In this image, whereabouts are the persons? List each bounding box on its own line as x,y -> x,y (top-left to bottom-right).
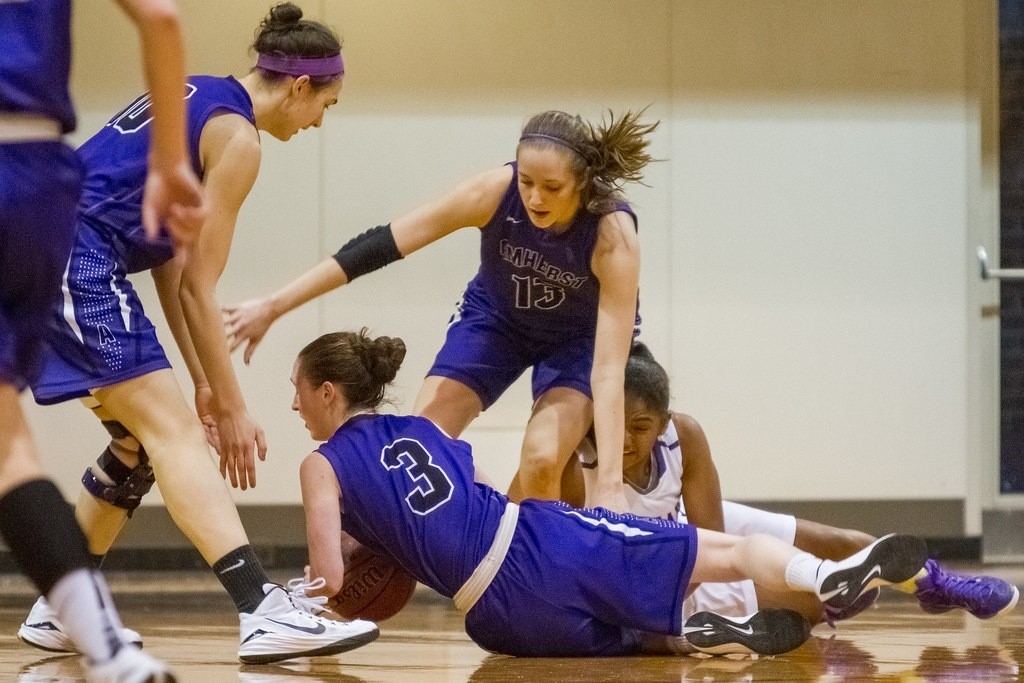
0,0 -> 207,683
220,112 -> 660,512
15,2 -> 380,664
508,343 -> 1021,624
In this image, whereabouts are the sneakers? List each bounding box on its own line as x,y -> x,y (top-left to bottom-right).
236,576 -> 379,665
76,643 -> 179,683
683,607 -> 810,660
16,592 -> 143,653
916,559 -> 1019,620
816,530 -> 929,619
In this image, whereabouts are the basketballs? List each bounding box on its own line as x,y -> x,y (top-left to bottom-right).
327,530 -> 418,620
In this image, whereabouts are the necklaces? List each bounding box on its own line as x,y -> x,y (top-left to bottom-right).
290,333 -> 928,657
